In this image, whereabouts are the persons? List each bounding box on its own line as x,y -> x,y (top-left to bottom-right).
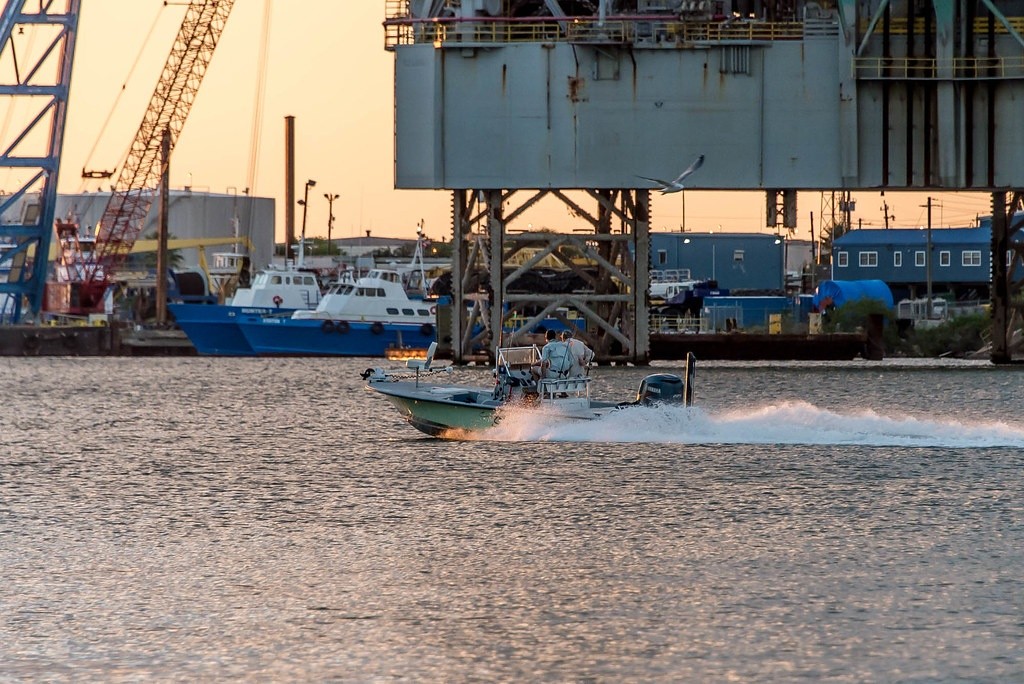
530,329 -> 595,399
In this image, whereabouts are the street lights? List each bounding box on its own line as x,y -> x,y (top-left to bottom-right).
296,179 -> 317,235
322,193 -> 340,257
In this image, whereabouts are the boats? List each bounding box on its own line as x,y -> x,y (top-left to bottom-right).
166,231 -> 325,356
228,220 -> 591,359
356,338 -> 686,434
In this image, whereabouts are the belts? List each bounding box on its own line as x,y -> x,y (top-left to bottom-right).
549,368 -> 568,373
